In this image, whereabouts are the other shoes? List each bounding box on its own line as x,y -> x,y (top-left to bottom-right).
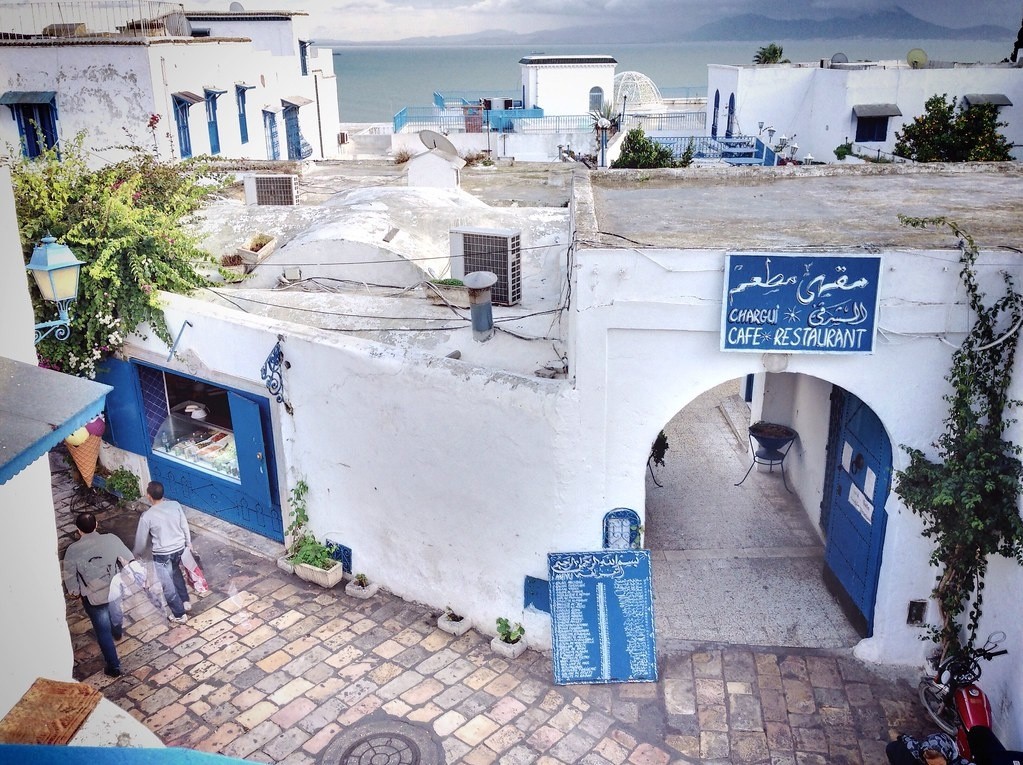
167,602 -> 193,624
104,631 -> 122,676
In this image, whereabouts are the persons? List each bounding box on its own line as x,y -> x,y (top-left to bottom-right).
131,481 -> 194,624
64,513 -> 145,675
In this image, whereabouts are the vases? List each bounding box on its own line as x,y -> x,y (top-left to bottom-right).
90,468 -> 115,492
747,421 -> 798,461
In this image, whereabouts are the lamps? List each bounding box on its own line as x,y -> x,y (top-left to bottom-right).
622,90 -> 629,97
757,119 -> 815,166
605,120 -> 611,129
23,237 -> 90,347
598,117 -> 607,130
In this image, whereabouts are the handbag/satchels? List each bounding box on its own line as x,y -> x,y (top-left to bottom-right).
182,547 -> 208,594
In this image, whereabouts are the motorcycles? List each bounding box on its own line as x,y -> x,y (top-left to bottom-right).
917,631 -> 1016,765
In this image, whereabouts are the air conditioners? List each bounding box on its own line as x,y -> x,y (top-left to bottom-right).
242,173 -> 301,207
340,131 -> 349,145
449,225 -> 524,306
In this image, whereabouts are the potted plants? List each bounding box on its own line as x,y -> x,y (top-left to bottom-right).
236,232 -> 278,265
61,450 -> 84,483
345,572 -> 378,599
292,531 -> 343,589
490,617 -> 528,659
219,253 -> 251,283
102,465 -> 143,510
437,605 -> 472,637
276,474 -> 315,574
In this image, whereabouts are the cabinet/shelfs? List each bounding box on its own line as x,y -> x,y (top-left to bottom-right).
152,412 -> 241,485
